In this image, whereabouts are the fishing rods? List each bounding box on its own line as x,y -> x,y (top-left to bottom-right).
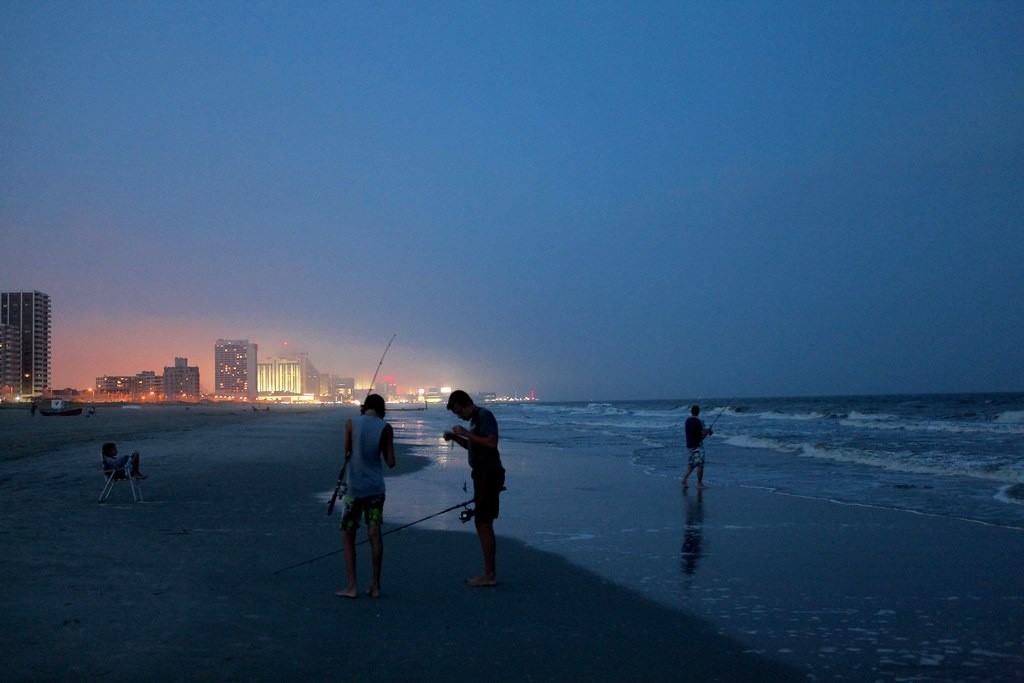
323,331 -> 399,517
705,392 -> 738,437
271,486 -> 510,576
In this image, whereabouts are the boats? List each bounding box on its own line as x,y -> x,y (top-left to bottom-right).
40,408 -> 82,416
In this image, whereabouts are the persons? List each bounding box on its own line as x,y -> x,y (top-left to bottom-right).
334,394 -> 396,597
31,404 -> 37,414
443,390 -> 506,586
681,405 -> 713,489
102,443 -> 148,479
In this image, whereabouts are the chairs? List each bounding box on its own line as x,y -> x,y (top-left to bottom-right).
98,443 -> 144,503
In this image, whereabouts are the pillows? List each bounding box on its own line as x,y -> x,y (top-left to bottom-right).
105,455 -> 129,468
125,448 -> 139,477
